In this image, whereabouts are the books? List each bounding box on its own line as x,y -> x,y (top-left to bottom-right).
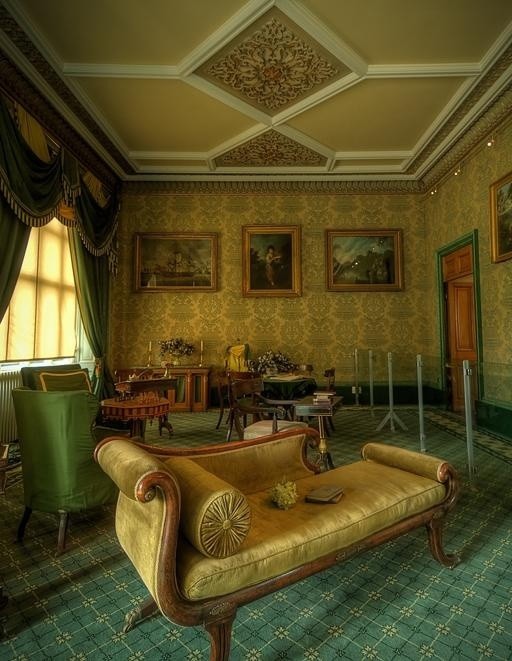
312,390 -> 338,405
328,491 -> 343,504
303,483 -> 344,503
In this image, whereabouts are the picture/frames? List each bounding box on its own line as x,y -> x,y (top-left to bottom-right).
488,169 -> 512,266
323,227 -> 409,296
239,224 -> 304,300
131,229 -> 220,295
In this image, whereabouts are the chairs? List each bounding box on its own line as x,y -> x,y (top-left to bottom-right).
10,364 -> 126,556
211,362 -> 338,442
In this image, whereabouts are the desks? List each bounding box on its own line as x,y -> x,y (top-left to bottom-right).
291,394 -> 346,472
99,378 -> 181,445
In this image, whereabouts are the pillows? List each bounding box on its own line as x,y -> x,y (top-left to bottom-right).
160,455 -> 253,561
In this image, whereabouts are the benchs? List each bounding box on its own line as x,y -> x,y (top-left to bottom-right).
89,428 -> 465,661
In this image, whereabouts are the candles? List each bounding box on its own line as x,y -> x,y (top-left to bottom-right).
200,340 -> 203,352
149,341 -> 152,352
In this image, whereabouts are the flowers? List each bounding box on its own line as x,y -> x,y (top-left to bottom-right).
247,351 -> 295,373
157,337 -> 196,360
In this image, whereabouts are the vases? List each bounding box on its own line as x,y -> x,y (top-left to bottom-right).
172,357 -> 181,366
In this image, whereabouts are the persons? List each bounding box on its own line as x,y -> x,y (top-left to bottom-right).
265,244 -> 282,288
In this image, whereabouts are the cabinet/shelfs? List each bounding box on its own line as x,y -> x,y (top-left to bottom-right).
131,366 -> 212,414
